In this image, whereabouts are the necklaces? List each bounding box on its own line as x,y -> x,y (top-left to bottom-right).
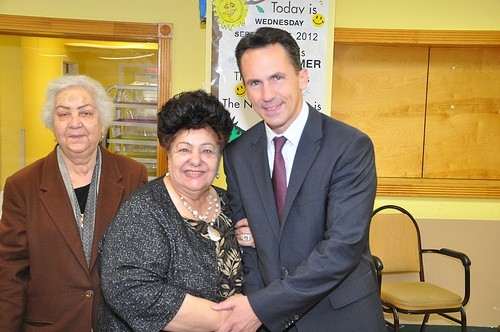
169,176 -> 219,226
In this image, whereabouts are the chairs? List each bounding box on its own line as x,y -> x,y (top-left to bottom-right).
367,204 -> 471,332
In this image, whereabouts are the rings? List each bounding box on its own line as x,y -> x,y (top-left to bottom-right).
243,233 -> 249,240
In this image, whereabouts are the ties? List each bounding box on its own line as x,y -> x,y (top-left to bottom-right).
272,137 -> 287,224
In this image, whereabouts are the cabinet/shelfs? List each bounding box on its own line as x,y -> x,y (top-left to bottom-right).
101,86 -> 157,182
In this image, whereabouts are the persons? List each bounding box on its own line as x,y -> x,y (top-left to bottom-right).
210,27 -> 388,332
96,89 -> 256,332
0,75 -> 148,332
